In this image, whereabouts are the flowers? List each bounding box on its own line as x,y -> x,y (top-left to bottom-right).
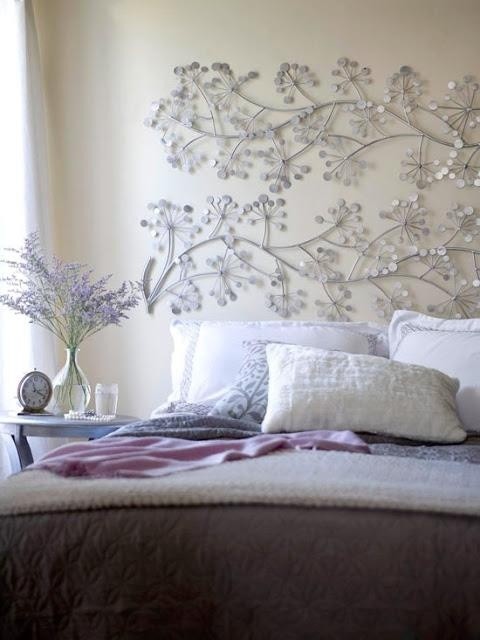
0,232 -> 142,347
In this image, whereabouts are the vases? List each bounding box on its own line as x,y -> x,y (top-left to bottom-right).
53,347 -> 91,412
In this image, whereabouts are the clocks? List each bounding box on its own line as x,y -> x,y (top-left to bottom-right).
17,368 -> 53,417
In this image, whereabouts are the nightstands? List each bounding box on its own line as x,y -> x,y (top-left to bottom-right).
1,413 -> 139,472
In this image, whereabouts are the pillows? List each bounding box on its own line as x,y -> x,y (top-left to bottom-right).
388,310 -> 480,432
149,318 -> 387,419
208,340 -> 283,424
261,343 -> 466,446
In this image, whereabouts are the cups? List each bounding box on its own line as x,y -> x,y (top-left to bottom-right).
96,380 -> 120,421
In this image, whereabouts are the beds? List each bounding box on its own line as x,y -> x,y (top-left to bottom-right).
0,412 -> 480,640
